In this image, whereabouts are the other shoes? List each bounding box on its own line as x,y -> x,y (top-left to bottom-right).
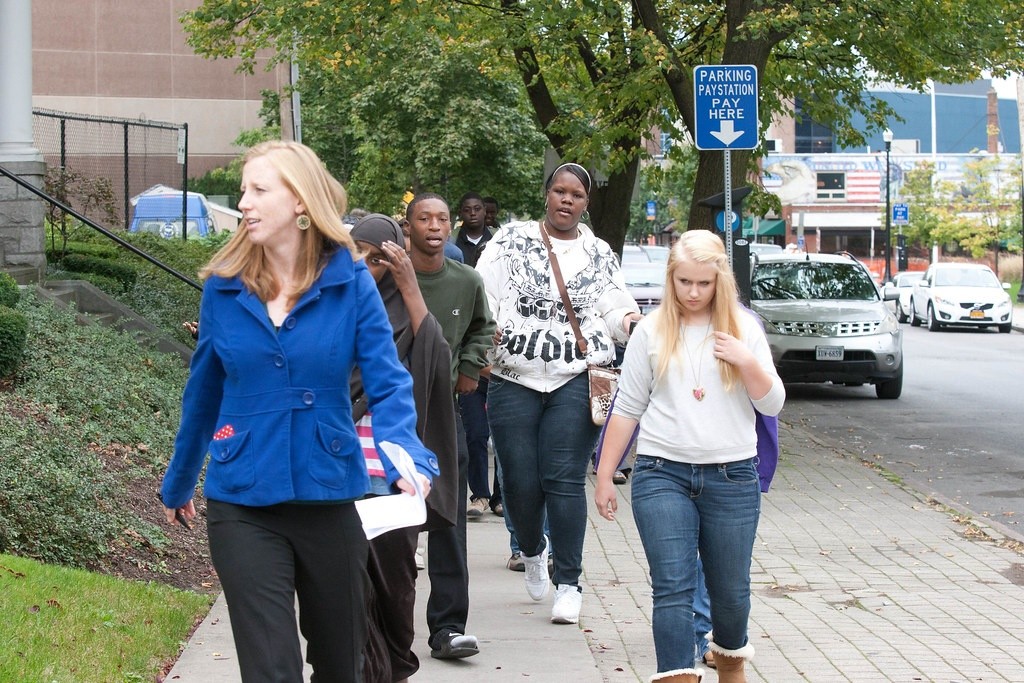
414,552 -> 426,569
612,470 -> 626,484
704,651 -> 716,668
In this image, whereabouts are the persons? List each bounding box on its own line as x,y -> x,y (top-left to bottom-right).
404,195 -> 498,658
398,191 -> 506,518
156,141 -> 437,683
594,228 -> 787,683
476,162 -> 648,623
595,303 -> 778,671
181,212 -> 454,683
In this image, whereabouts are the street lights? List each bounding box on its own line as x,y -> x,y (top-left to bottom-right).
882,127 -> 893,286
924,79 -> 938,263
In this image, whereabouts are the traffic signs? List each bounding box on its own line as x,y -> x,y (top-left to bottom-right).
693,65 -> 759,150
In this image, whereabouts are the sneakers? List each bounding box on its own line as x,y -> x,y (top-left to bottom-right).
494,503 -> 504,517
550,583 -> 582,624
467,496 -> 490,518
548,552 -> 555,579
520,534 -> 549,601
506,554 -> 525,572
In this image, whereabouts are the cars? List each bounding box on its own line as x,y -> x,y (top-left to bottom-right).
909,262 -> 1013,333
878,272 -> 926,323
749,244 -> 782,255
620,245 -> 673,316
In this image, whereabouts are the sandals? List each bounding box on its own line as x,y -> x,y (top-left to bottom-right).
430,629 -> 479,660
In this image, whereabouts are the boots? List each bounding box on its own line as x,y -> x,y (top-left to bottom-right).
705,628 -> 755,683
649,667 -> 705,683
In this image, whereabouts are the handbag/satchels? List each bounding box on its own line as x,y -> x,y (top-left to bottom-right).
587,364 -> 621,426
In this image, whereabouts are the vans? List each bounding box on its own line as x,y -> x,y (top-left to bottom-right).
131,191 -> 218,236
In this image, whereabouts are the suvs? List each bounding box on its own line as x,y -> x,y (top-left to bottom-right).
749,251 -> 904,398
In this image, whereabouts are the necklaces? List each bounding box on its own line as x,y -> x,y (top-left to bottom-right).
542,217 -> 579,259
679,305 -> 712,403
